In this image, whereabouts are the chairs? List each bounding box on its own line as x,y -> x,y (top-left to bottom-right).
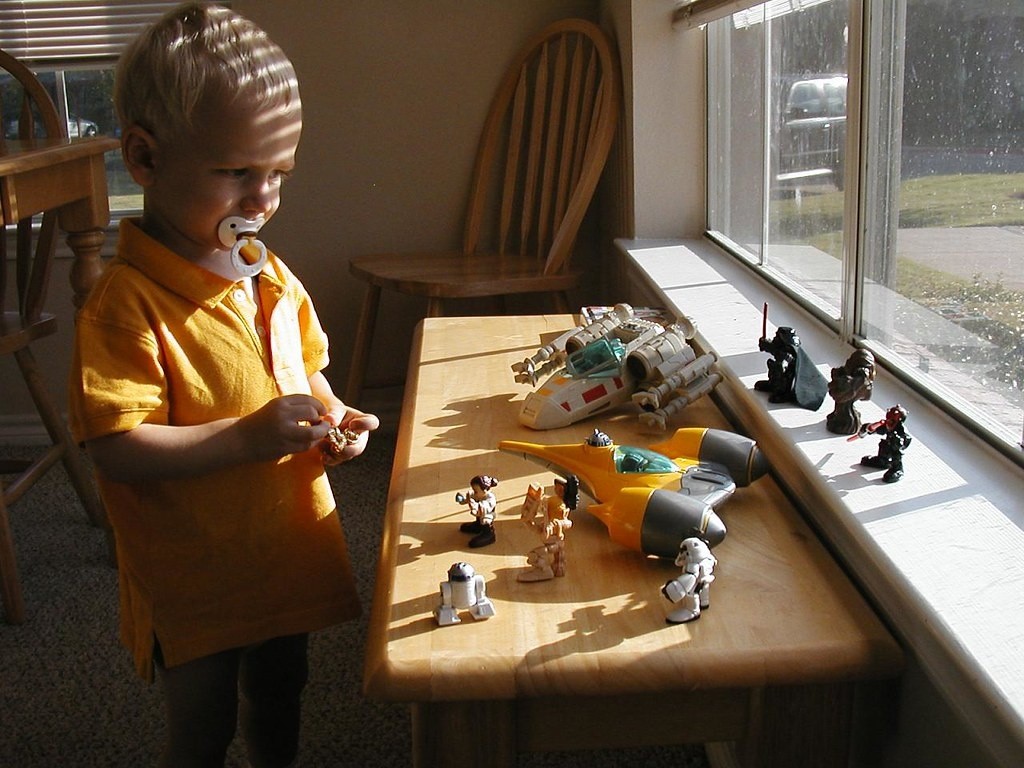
345,19 -> 618,437
0,48 -> 125,624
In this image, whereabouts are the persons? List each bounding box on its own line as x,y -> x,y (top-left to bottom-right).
455,475 -> 498,548
517,474 -> 579,582
754,299 -> 913,482
661,537 -> 717,625
63,0 -> 381,768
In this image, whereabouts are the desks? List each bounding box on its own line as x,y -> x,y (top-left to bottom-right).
0,132 -> 122,310
366,314 -> 896,768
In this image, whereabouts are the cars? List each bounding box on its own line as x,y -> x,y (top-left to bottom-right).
776,76 -> 851,188
6,111 -> 103,140
